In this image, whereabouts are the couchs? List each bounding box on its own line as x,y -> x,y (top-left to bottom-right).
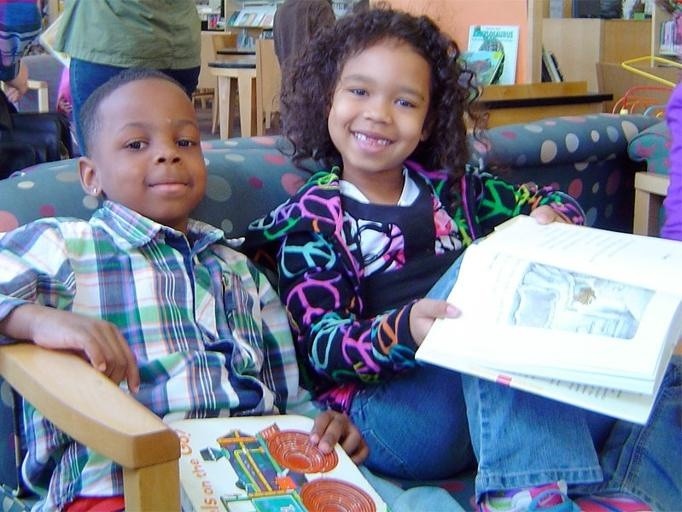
1,112 -> 658,512
13,55 -> 66,114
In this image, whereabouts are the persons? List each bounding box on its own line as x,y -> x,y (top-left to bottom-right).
244,10 -> 681,512
273,1 -> 336,68
0,0 -> 201,179
660,84 -> 682,239
0,70 -> 461,511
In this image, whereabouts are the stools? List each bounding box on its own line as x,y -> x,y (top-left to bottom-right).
208,60 -> 259,140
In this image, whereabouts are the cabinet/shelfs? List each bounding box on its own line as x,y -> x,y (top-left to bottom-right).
217,0 -> 282,132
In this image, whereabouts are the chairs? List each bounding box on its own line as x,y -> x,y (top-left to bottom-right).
1,83 -> 66,179
629,119 -> 674,237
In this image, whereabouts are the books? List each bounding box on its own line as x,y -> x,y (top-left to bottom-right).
416,210 -> 682,426
163,414 -> 388,511
456,25 -> 519,101
